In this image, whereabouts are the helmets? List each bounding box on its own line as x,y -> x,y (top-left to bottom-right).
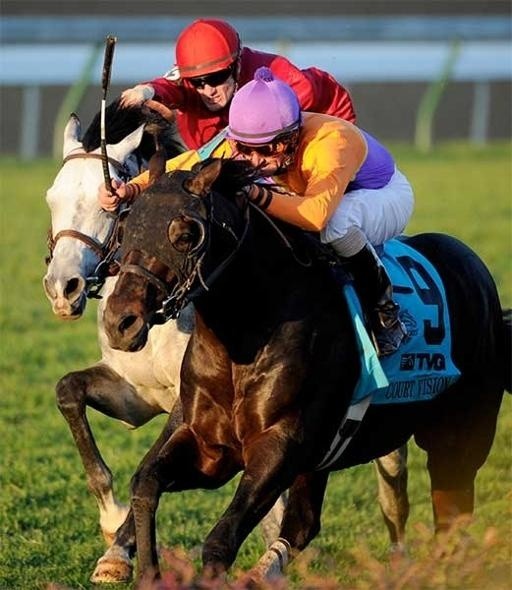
175,15 -> 242,78
224,67 -> 302,149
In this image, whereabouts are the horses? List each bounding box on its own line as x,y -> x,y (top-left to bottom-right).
41,113 -> 412,548
100,148 -> 512,589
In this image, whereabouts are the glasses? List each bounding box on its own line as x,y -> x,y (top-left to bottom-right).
235,145 -> 274,158
188,65 -> 235,90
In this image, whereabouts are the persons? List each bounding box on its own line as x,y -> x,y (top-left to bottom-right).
96,64 -> 416,359
115,11 -> 360,154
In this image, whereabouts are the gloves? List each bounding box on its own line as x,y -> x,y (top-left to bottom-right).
119,83 -> 157,107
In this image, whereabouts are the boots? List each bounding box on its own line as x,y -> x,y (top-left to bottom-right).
342,241 -> 409,357
